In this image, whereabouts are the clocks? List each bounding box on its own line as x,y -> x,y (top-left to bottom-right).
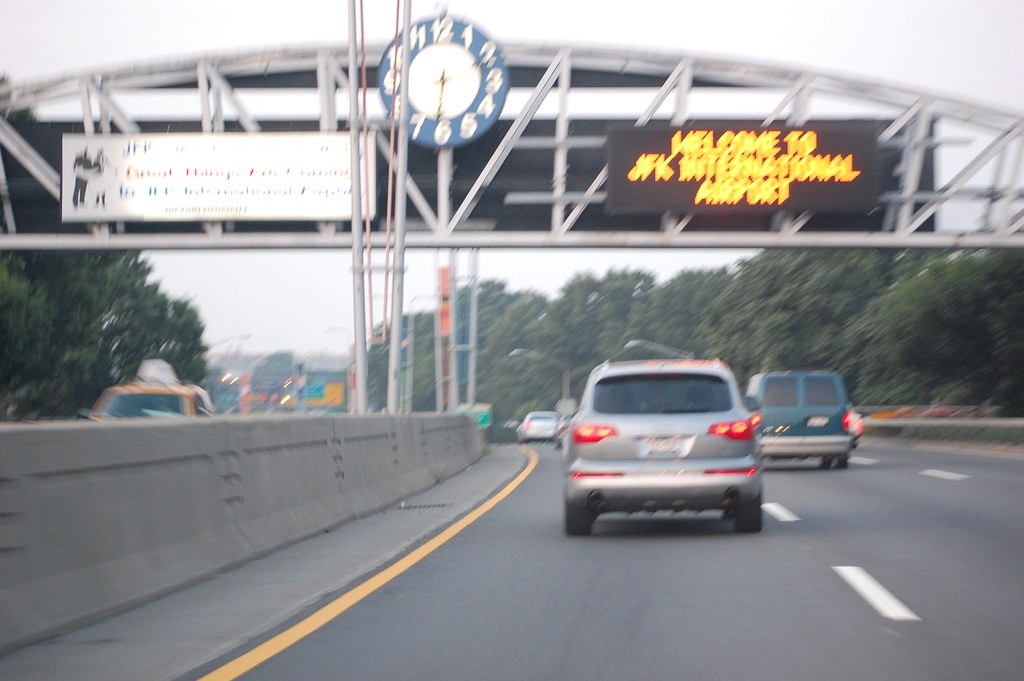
375,17 -> 511,150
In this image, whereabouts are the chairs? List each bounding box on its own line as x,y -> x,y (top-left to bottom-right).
600,384 -> 642,414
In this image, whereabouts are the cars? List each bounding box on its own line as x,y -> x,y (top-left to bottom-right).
743,369 -> 864,471
76,358 -> 217,423
516,410 -> 559,444
553,355 -> 766,537
